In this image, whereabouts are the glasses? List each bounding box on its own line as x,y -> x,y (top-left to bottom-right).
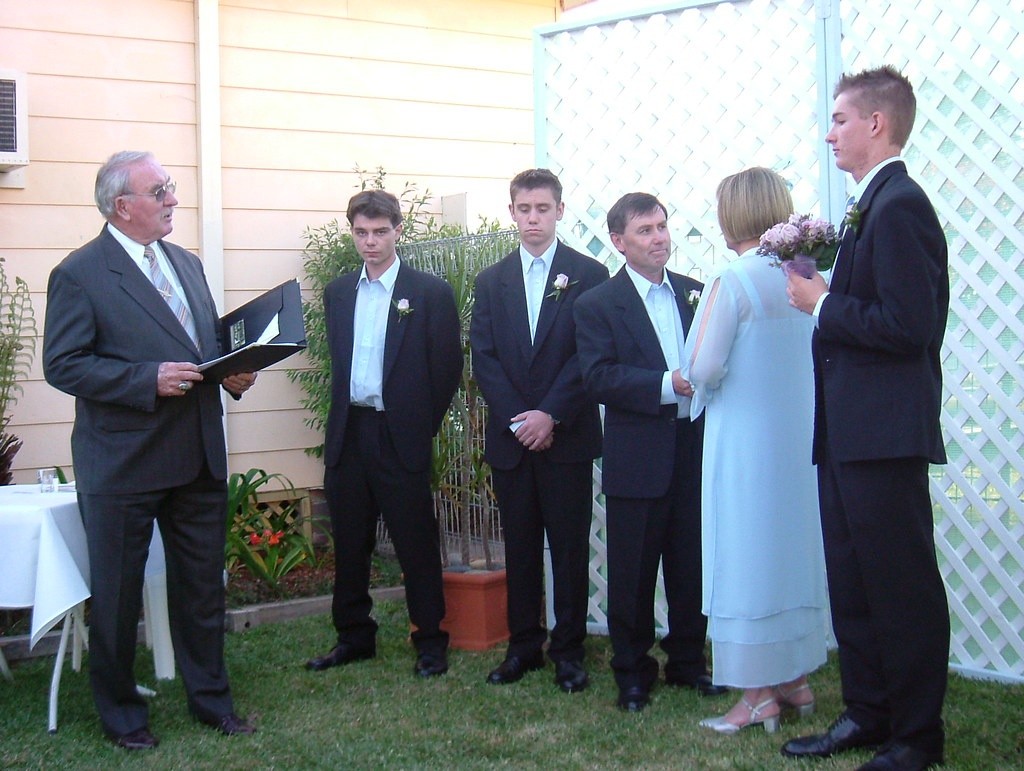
121,182 -> 176,203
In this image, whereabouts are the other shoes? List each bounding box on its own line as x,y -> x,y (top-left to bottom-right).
110,727 -> 158,752
200,713 -> 256,739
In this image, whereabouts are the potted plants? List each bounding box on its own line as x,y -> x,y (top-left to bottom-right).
402,244 -> 510,650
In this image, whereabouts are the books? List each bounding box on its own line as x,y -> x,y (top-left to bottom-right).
196,278 -> 308,384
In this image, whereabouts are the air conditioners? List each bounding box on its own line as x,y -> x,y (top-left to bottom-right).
0,72 -> 30,173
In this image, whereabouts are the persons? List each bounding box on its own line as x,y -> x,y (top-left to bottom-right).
303,190 -> 463,675
43,151 -> 257,750
470,168 -> 609,691
782,66 -> 951,771
573,192 -> 727,711
677,167 -> 837,735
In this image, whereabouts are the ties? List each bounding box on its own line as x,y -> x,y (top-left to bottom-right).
144,246 -> 203,357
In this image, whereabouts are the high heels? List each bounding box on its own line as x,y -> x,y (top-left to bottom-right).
775,682 -> 814,719
699,696 -> 780,734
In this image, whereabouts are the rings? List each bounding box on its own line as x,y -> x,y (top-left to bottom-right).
179,382 -> 189,391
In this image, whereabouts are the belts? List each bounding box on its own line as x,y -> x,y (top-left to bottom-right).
349,405 -> 385,416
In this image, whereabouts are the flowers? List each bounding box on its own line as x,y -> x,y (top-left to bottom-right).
684,288 -> 701,313
845,203 -> 861,233
546,273 -> 580,301
391,298 -> 414,323
755,213 -> 842,279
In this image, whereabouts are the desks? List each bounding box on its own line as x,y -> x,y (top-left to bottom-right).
0,485 -> 175,734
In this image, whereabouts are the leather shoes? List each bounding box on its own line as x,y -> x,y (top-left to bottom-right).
414,646 -> 448,679
305,643 -> 376,671
486,653 -> 545,685
665,669 -> 725,696
779,711 -> 890,759
555,658 -> 588,694
853,736 -> 944,771
618,683 -> 648,713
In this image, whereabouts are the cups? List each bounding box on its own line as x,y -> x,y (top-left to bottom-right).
38,468 -> 59,493
